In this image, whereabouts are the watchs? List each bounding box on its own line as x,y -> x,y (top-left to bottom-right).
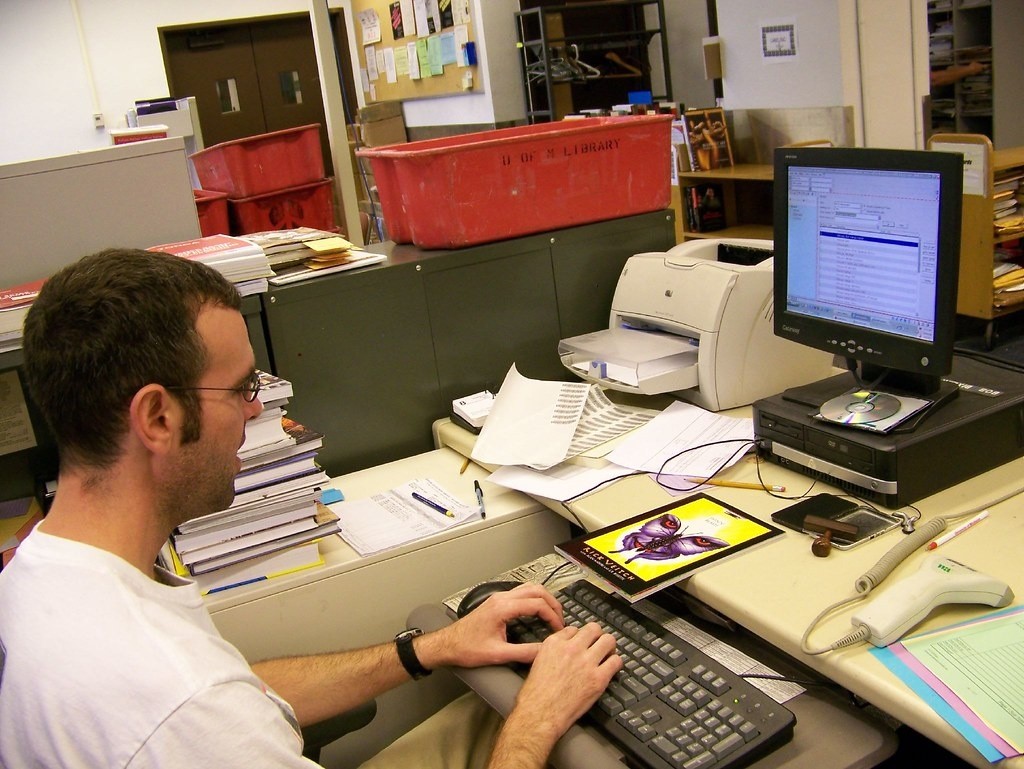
395,628 -> 433,681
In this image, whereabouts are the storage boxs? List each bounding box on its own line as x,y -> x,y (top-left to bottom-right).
187,123 -> 336,239
348,101 -> 674,251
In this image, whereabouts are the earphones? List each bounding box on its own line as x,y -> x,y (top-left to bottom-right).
892,511 -> 910,527
902,516 -> 918,535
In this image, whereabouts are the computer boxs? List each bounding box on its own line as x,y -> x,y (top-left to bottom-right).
752,353 -> 1024,510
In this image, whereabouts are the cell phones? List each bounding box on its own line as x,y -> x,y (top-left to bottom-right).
769,493 -> 859,532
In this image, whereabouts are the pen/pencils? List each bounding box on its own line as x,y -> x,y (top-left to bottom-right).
924,509 -> 991,552
474,479 -> 488,519
411,491 -> 456,519
682,476 -> 786,492
457,456 -> 473,476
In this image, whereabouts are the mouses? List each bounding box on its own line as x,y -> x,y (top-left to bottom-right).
457,581 -> 524,619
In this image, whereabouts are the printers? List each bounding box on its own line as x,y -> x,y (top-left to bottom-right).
558,237 -> 849,412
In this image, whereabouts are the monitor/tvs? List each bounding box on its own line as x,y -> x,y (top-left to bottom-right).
771,146 -> 964,432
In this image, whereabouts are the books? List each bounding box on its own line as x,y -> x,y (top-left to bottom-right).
148,226 -> 388,296
929,0 -> 992,119
993,262 -> 1020,277
992,181 -> 1019,219
554,494 -> 786,603
169,367 -> 341,594
109,125 -> 167,145
0,279 -> 49,354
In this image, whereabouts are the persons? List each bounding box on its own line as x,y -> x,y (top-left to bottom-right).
0,247 -> 621,769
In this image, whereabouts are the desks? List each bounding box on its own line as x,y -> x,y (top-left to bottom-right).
0,206 -> 1024,767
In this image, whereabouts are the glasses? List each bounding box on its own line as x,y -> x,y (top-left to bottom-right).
128,370 -> 263,402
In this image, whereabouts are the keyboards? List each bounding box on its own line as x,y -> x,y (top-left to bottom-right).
505,578 -> 797,769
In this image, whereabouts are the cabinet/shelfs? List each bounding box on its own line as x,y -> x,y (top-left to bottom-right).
927,0 -> 1024,150
674,172 -> 777,249
928,133 -> 1024,349
513,0 -> 674,124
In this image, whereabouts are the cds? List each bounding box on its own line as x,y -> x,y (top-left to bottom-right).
819,392 -> 901,423
